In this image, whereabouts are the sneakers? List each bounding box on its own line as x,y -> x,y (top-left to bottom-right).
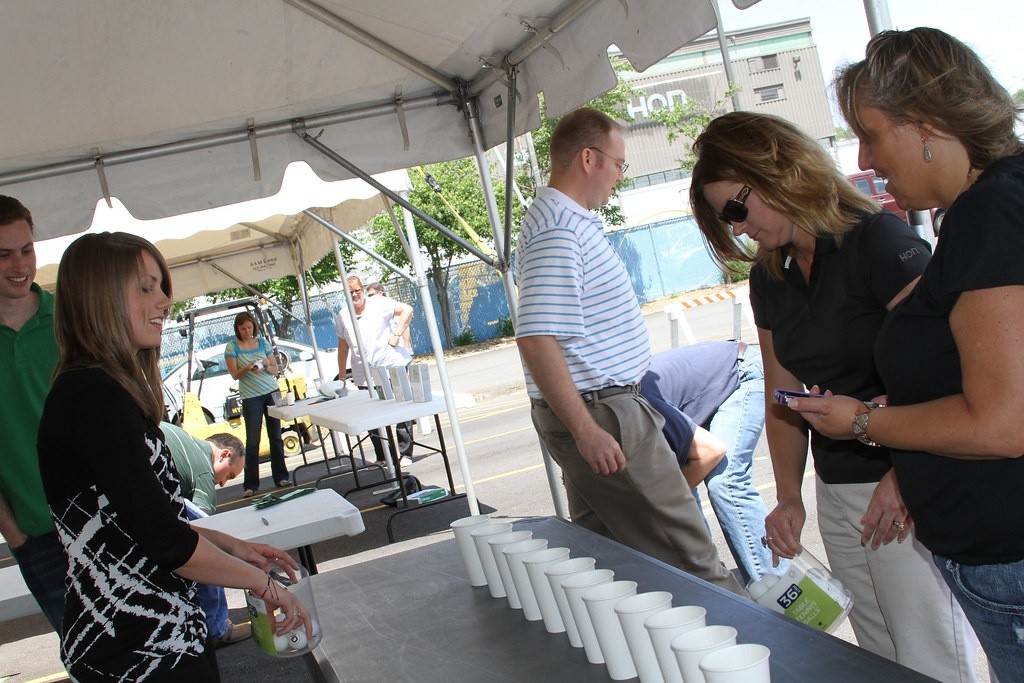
212,619 -> 252,649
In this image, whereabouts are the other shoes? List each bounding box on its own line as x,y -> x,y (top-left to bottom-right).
277,480 -> 291,487
243,488 -> 256,498
399,455 -> 412,467
374,460 -> 387,468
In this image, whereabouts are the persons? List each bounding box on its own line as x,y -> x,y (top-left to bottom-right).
36,231 -> 313,683
790,27 -> 1024,683
366,282 -> 414,356
514,107 -> 746,597
335,276 -> 413,467
158,421 -> 253,648
688,111 -> 991,683
0,194 -> 65,639
224,312 -> 292,497
636,339 -> 790,587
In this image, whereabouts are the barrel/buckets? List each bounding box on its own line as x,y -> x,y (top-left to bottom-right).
743,544 -> 856,635
243,562 -> 322,657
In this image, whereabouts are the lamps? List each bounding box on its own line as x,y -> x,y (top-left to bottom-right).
793,55 -> 800,70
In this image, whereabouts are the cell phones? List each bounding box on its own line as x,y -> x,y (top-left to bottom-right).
771,389 -> 825,406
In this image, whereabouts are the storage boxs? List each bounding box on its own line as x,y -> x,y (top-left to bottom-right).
396,489 -> 448,508
371,364 -> 432,403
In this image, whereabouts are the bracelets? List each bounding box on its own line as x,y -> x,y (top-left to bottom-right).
394,334 -> 401,336
248,573 -> 279,600
852,401 -> 885,447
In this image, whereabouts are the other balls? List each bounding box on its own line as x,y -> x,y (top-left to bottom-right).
272,613 -> 319,652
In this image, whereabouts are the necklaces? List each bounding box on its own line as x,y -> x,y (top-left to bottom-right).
933,166 -> 973,238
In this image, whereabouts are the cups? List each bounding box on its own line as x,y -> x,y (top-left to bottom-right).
314,377 -> 325,395
450,515 -> 491,587
545,557 -> 596,648
470,523 -> 514,598
671,625 -> 737,683
522,547 -> 570,633
698,643 -> 771,683
614,592 -> 673,683
644,606 -> 706,683
582,580 -> 638,680
488,531 -> 533,608
503,539 -> 548,621
561,570 -> 614,664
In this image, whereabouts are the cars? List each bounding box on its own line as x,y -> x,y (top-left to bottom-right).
159,337 -> 367,429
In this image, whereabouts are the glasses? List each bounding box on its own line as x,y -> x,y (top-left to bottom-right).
350,288 -> 362,295
368,292 -> 379,297
719,184 -> 752,226
586,146 -> 629,173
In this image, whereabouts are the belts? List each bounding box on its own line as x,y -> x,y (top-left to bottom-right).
735,341 -> 747,366
534,382 -> 641,408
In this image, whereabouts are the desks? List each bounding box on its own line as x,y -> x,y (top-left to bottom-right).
286,515 -> 944,683
0,488 -> 366,623
309,390 -> 482,544
268,391 -> 387,490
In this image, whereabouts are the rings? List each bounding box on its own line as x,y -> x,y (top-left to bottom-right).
761,536 -> 772,549
893,521 -> 905,530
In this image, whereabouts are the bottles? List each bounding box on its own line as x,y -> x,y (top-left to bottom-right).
251,361 -> 262,371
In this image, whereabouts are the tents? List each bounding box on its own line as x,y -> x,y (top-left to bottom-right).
0,0 -> 762,523
33,161 -> 480,517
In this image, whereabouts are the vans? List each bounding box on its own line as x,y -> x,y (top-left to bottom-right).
841,169 -> 946,239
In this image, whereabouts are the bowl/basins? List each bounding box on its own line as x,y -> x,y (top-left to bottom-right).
271,389 -> 295,407
319,381 -> 344,397
